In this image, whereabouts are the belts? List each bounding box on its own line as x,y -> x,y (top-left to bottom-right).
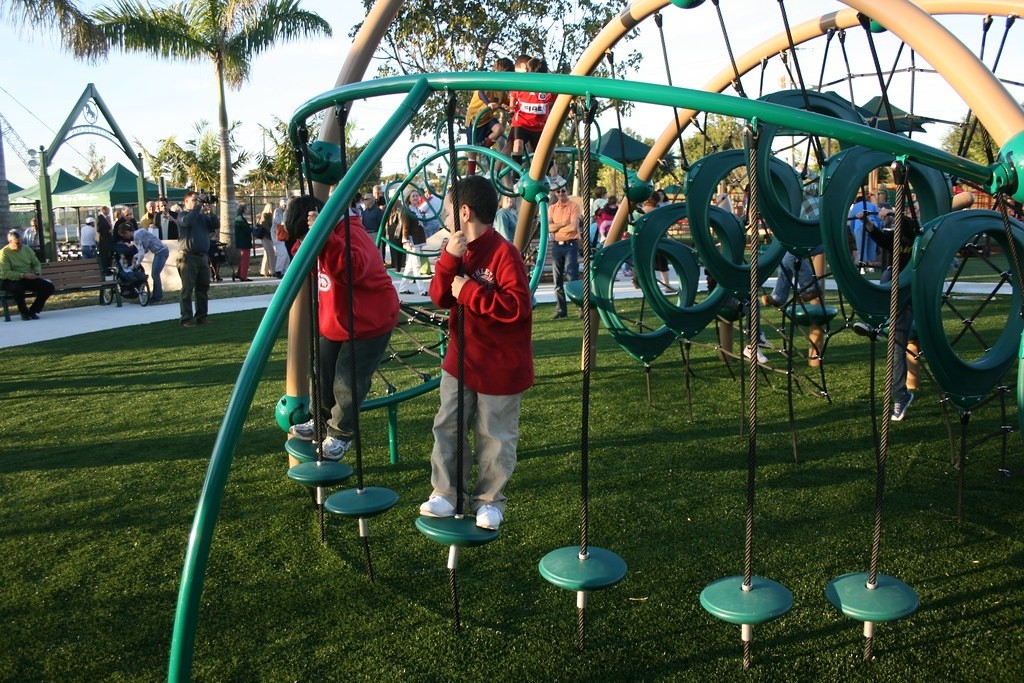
555,239 -> 577,246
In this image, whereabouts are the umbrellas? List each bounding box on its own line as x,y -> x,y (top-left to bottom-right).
858,96 -> 928,134
579,129 -> 670,199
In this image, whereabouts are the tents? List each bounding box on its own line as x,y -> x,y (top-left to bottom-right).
7,161 -> 195,223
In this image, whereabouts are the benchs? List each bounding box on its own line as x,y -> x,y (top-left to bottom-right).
526,238 -> 585,283
0,257 -> 123,322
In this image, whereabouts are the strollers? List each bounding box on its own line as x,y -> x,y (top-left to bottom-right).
208,236 -> 236,282
102,242 -> 150,306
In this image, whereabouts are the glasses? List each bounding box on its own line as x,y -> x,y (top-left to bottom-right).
556,190 -> 566,193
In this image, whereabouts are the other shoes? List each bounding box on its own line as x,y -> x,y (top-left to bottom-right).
201,319 -> 210,323
421,291 -> 429,296
548,310 -> 567,320
21,310 -> 29,320
800,291 -> 817,304
149,297 -> 156,302
400,290 -> 415,294
28,310 -> 39,319
577,309 -> 584,318
183,321 -> 196,327
762,295 -> 780,307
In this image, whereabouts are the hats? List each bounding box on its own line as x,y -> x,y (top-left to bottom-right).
86,217 -> 95,223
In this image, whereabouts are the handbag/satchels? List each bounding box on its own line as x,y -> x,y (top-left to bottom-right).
419,246 -> 431,274
252,224 -> 266,239
277,225 -> 289,241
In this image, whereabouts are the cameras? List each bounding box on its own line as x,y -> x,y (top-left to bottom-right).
201,189 -> 218,203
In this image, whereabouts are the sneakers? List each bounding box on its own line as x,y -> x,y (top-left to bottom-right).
475,504 -> 503,530
289,419 -> 327,440
758,335 -> 771,348
420,496 -> 456,518
891,393 -> 914,421
315,437 -> 351,459
743,346 -> 768,363
853,322 -> 883,341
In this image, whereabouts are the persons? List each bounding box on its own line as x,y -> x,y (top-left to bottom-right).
418,174 -> 534,530
711,164 -> 976,420
81,54 -> 682,321
175,190 -> 221,328
0,228 -> 55,321
283,194 -> 400,461
